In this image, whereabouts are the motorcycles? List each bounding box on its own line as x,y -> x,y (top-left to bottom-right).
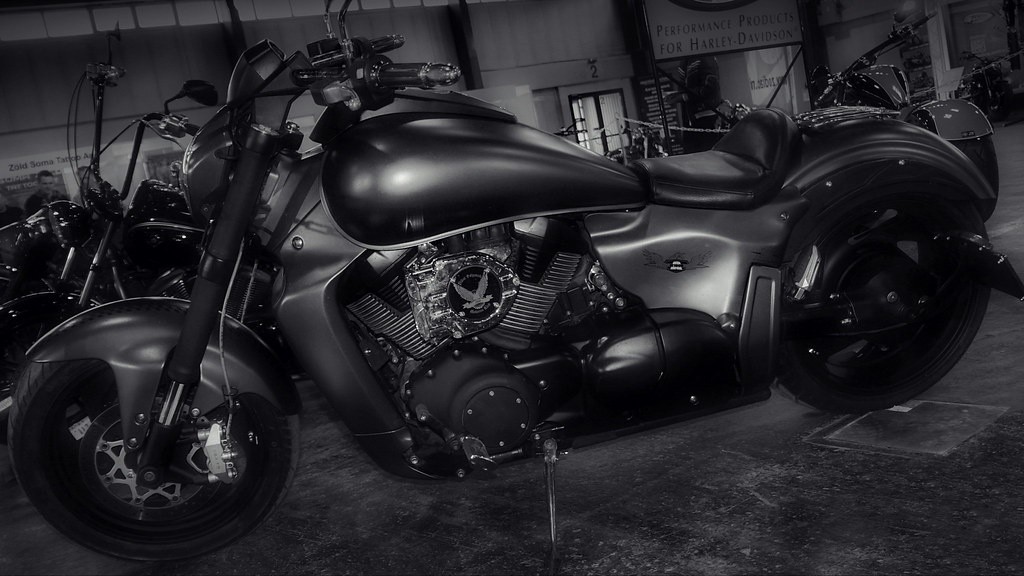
0,23 -> 1024,460
8,1 -> 1023,562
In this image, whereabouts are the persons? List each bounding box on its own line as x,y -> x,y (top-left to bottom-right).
0,166 -> 104,229
665,55 -> 722,154
